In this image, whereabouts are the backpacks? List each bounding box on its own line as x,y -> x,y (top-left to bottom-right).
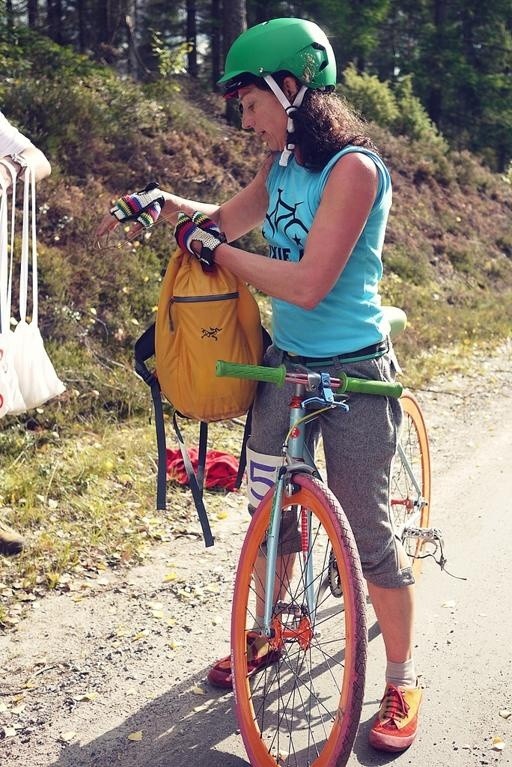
154,236 -> 265,421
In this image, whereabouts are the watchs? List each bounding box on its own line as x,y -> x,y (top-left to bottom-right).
3,153 -> 29,178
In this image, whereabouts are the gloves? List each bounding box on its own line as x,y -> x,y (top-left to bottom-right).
110,182 -> 167,231
168,207 -> 230,269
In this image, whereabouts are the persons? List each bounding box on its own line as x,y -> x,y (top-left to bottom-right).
96,18 -> 422,752
0,109 -> 51,554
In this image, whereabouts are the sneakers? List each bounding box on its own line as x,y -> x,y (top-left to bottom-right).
209,625 -> 276,693
367,677 -> 425,754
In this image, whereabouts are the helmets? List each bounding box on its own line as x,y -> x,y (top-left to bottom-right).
211,17 -> 338,97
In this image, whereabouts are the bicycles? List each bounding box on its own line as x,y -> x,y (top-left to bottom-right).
214,305 -> 467,767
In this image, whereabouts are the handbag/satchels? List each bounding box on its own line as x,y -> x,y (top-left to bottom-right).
0,315 -> 69,419
